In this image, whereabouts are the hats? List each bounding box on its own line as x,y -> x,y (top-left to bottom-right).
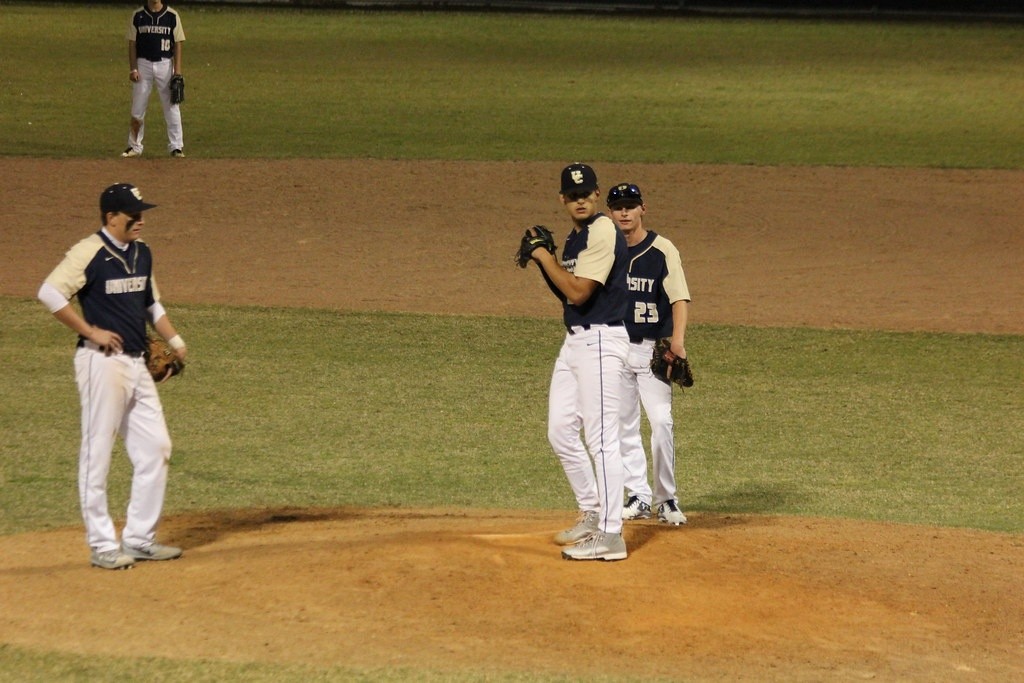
100,182 -> 157,214
561,163 -> 598,198
607,183 -> 642,208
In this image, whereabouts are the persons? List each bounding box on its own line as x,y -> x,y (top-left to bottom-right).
514,162 -> 631,562
605,182 -> 695,526
120,0 -> 186,159
37,181 -> 188,571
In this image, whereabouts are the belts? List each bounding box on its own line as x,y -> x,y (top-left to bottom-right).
78,338 -> 142,358
567,319 -> 625,334
145,56 -> 161,63
629,334 -> 643,345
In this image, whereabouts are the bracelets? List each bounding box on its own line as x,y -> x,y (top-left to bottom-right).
167,335 -> 186,350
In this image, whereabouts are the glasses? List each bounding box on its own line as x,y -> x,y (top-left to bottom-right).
608,186 -> 640,202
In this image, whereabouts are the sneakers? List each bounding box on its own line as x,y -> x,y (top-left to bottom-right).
91,545 -> 135,570
554,509 -> 601,545
561,528 -> 628,561
171,149 -> 185,158
120,542 -> 183,561
121,147 -> 138,158
620,496 -> 651,520
657,500 -> 688,526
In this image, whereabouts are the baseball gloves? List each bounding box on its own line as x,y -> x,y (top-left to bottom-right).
516,224 -> 557,268
651,339 -> 694,388
144,334 -> 185,382
168,74 -> 185,104
